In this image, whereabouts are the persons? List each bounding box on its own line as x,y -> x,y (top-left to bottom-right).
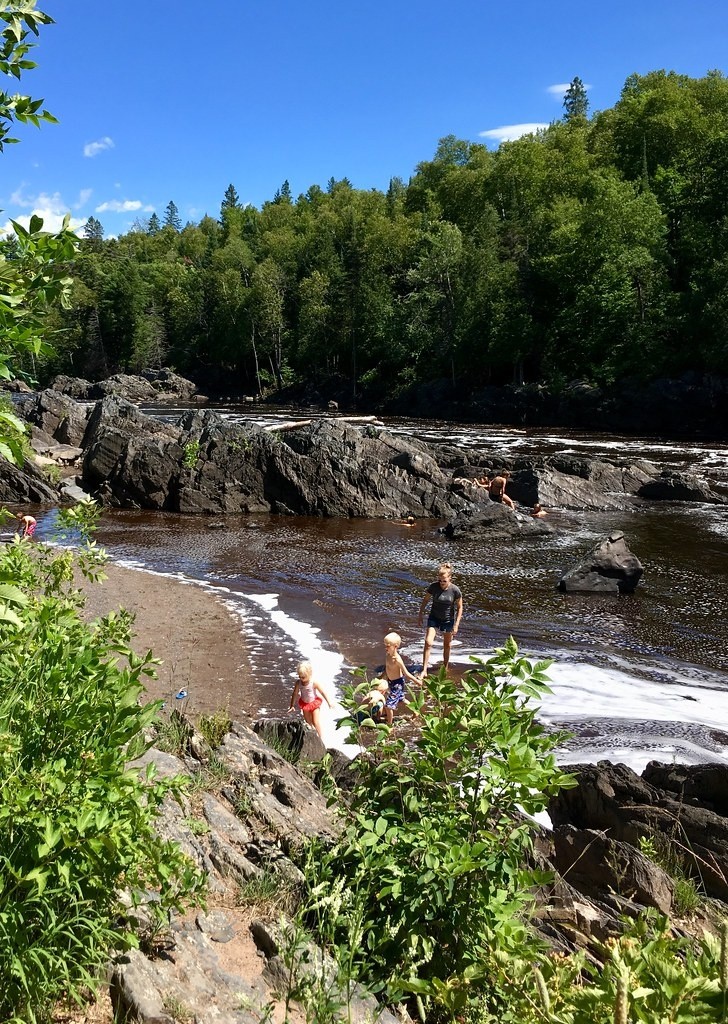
529,503 -> 549,518
416,566 -> 462,679
358,679 -> 388,726
16,512 -> 36,542
473,468 -> 516,510
287,664 -> 333,738
384,632 -> 422,725
